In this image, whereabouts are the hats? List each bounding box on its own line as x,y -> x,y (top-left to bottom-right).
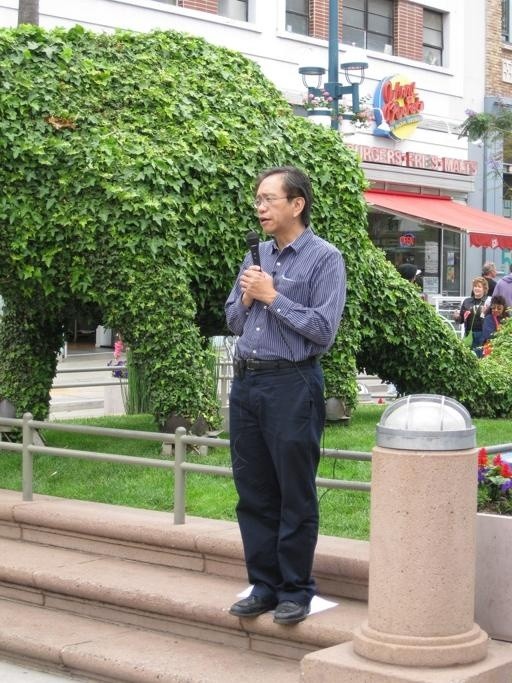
398,264 -> 417,278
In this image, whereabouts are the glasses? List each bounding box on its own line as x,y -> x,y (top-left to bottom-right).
255,195 -> 290,208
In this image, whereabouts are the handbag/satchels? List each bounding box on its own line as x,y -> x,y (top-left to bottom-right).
461,331 -> 473,346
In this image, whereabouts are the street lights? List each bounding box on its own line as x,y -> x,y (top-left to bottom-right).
298,46 -> 368,131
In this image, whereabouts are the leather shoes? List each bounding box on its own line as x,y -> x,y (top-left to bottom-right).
273,600 -> 311,624
228,595 -> 275,617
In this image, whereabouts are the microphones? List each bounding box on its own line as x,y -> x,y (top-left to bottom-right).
246,232 -> 261,272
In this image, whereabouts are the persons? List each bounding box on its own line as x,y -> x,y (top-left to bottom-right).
224,167 -> 347,625
452,261 -> 512,359
396,263 -> 426,303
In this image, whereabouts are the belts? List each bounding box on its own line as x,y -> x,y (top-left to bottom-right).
245,356 -> 320,370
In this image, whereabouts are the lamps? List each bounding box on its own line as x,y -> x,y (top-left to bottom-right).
370,108 -> 390,132
